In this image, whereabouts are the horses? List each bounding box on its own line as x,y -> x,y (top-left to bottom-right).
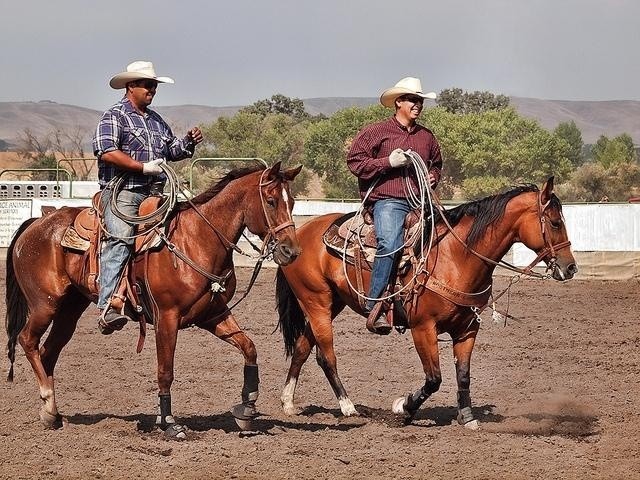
275,175 -> 579,432
5,161 -> 303,438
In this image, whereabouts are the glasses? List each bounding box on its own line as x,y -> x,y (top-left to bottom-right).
402,96 -> 423,104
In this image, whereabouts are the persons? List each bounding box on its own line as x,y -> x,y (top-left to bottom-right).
90,59 -> 205,336
344,76 -> 443,333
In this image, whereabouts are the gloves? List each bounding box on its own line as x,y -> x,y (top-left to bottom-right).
389,148 -> 412,168
143,158 -> 163,175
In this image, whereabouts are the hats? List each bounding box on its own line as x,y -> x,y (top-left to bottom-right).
380,77 -> 436,106
110,61 -> 174,89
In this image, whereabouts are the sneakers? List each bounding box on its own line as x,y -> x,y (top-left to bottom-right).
374,315 -> 388,328
99,313 -> 128,335
